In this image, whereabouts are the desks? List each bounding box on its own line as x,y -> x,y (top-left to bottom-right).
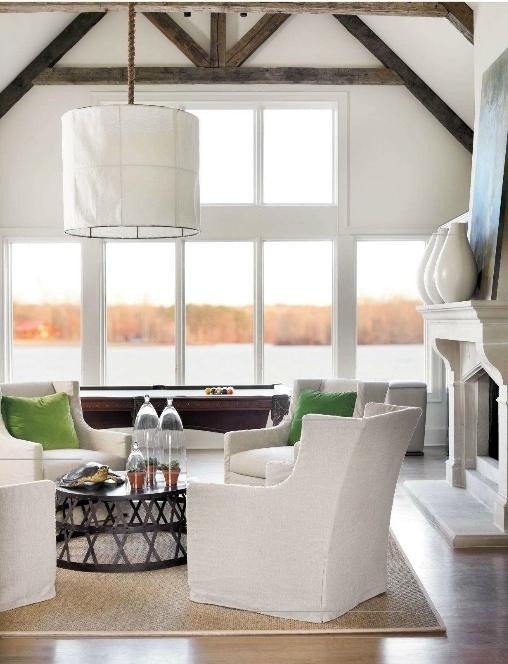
72,385 -> 276,438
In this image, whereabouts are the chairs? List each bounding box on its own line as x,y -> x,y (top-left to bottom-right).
0,378 -> 133,614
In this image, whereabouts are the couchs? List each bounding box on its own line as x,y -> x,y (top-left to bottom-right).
185,402 -> 424,623
223,374 -> 390,486
385,381 -> 427,453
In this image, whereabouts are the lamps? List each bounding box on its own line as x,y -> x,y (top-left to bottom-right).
60,1 -> 204,246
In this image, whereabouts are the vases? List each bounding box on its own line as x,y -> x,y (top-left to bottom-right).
414,221 -> 479,306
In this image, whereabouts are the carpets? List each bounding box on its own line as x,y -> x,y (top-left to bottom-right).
0,503 -> 448,639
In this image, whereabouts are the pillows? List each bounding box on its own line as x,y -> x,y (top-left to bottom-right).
286,387 -> 357,446
